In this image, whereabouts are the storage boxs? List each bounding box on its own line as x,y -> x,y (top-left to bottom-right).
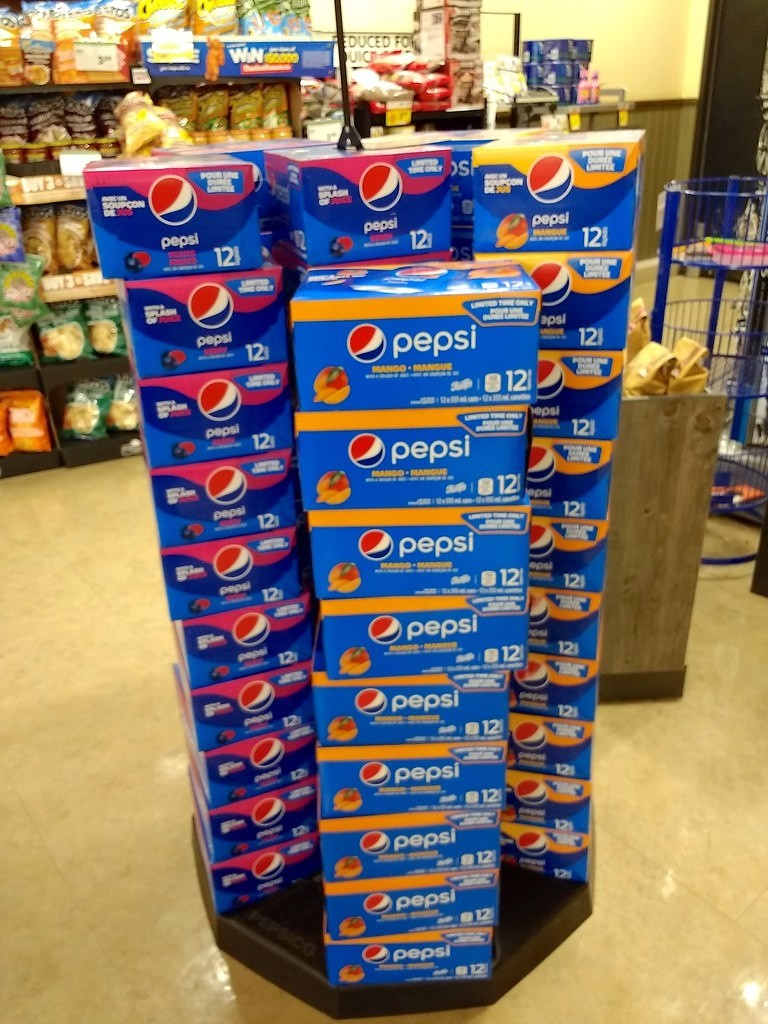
522,39 -> 593,105
413,0 -> 485,112
141,40 -> 338,79
81,128 -> 648,972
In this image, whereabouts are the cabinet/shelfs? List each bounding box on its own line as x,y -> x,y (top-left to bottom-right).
650,176 -> 768,566
0,77 -> 311,480
